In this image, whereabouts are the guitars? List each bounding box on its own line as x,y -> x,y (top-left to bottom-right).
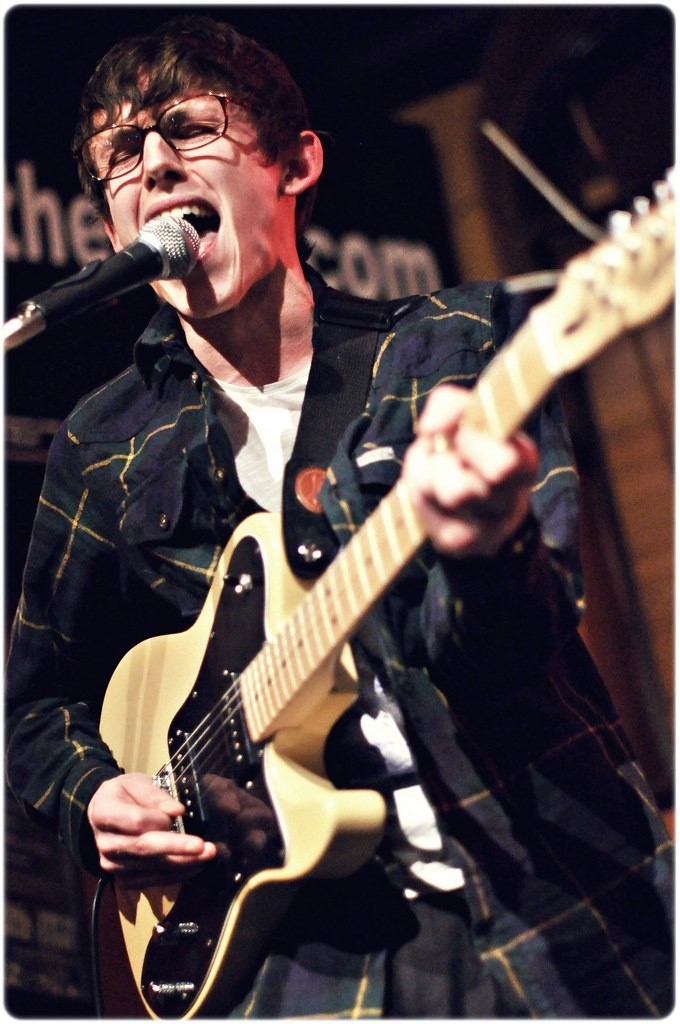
86,158 -> 675,1020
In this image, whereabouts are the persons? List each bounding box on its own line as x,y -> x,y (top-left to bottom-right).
3,21 -> 676,1019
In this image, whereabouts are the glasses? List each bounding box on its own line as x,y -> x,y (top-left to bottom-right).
76,94 -> 290,181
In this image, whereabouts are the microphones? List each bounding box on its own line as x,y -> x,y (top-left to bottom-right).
4,215 -> 201,351
